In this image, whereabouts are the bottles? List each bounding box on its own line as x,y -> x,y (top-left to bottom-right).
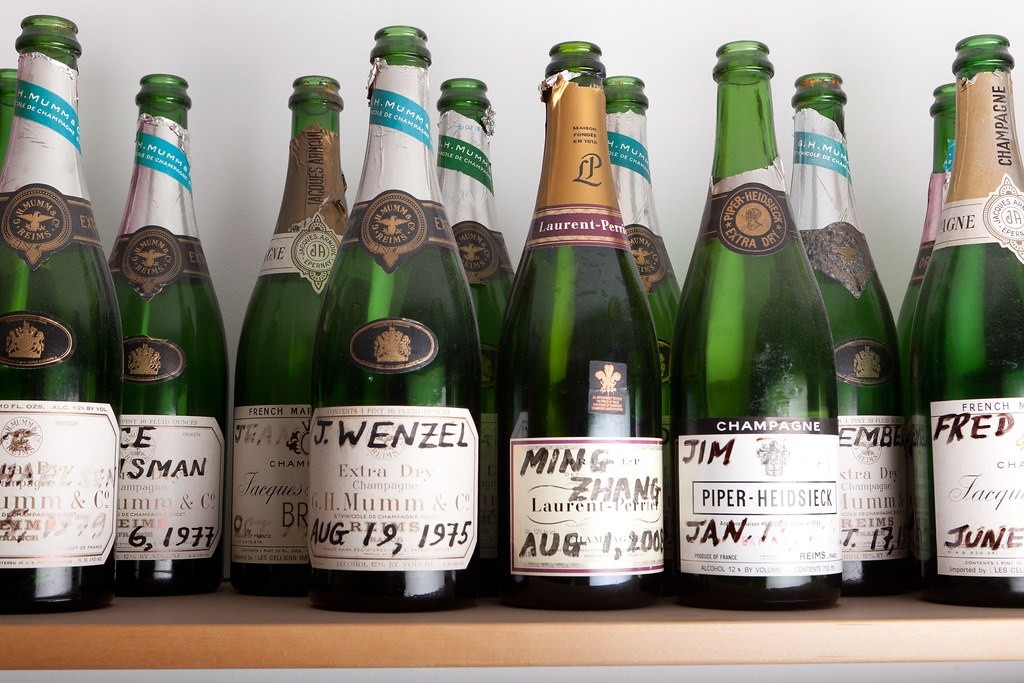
0,13 -> 1017,607
